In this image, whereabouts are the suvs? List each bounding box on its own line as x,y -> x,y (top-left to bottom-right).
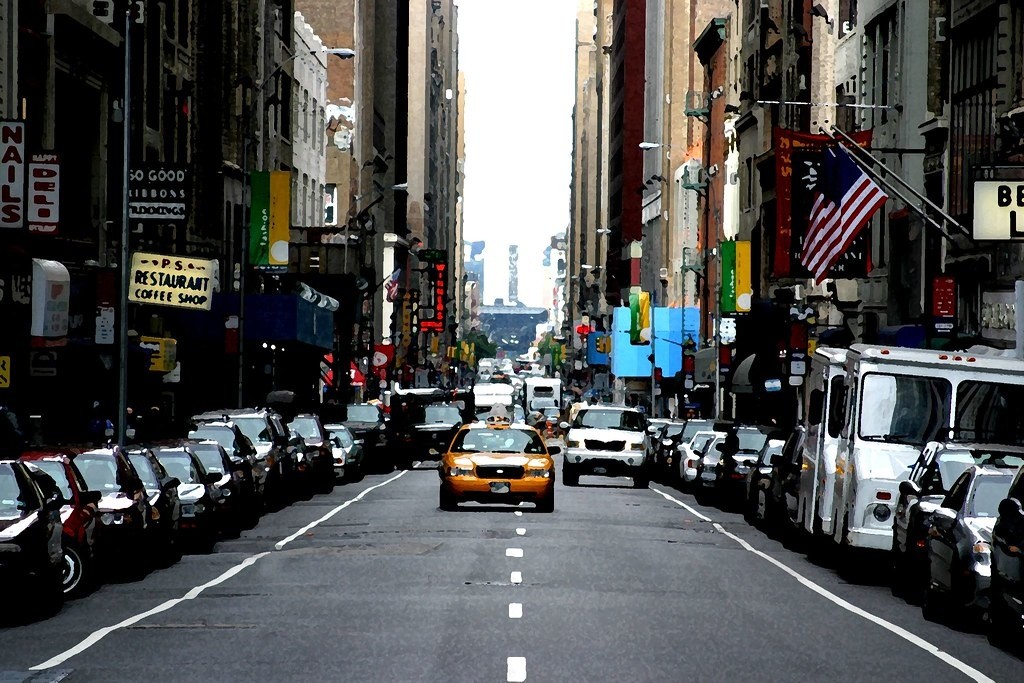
285,413 -> 333,495
187,414 -> 261,529
333,402 -> 390,474
559,404 -> 653,489
190,406 -> 290,511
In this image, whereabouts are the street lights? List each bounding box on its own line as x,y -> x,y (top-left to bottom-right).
229,45 -> 721,419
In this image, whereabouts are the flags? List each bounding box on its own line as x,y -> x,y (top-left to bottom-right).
384,270 -> 401,302
798,144 -> 887,286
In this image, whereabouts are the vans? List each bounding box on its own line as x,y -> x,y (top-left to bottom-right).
830,345 -> 1023,581
797,346 -> 848,563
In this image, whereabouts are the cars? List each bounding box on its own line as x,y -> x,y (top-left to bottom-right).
328,436 -> 348,486
323,424 -> 364,477
150,443 -> 223,553
437,417 -> 555,512
389,357 -> 580,472
167,438 -> 241,538
1,459 -> 64,618
58,440 -> 149,575
289,431 -> 315,500
121,446 -> 183,570
646,343 -> 1024,662
1,451 -> 101,600
